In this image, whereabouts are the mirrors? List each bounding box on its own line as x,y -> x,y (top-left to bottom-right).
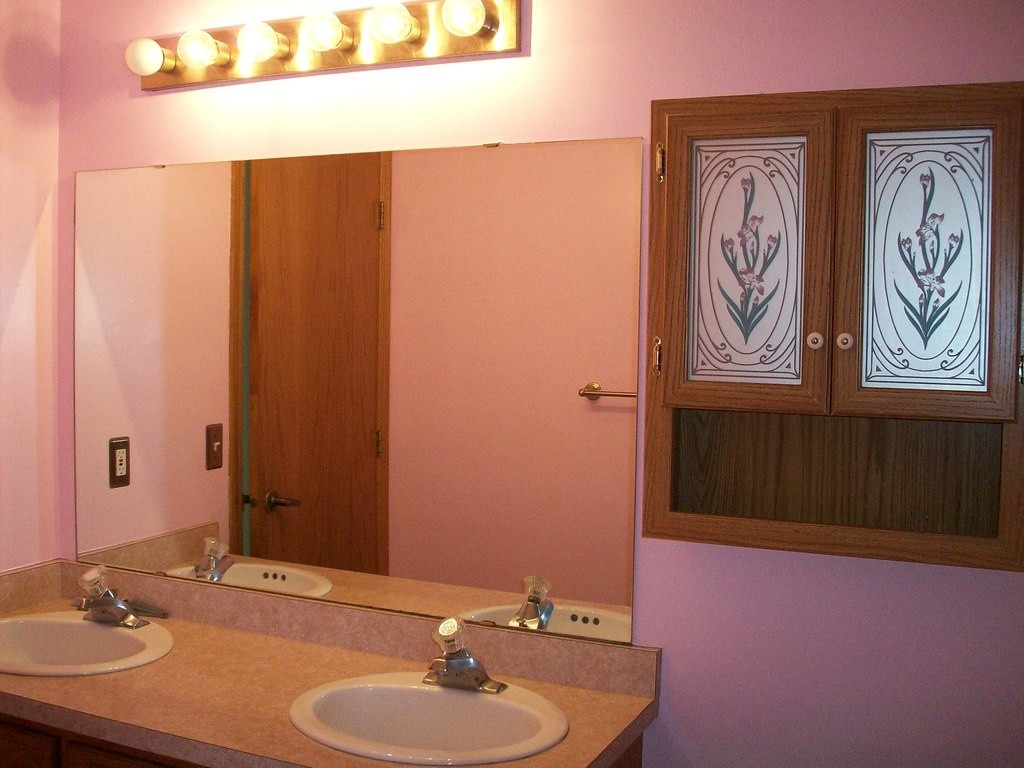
74,138 -> 640,643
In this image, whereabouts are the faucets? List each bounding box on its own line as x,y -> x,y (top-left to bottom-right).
508,576 -> 554,631
422,615 -> 508,694
195,537 -> 235,583
76,566 -> 151,630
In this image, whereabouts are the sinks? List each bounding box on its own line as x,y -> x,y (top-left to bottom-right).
0,611 -> 175,677
288,671 -> 570,764
462,605 -> 632,643
163,565 -> 334,598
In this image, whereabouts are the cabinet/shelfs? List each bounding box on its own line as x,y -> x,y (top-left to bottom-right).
651,81 -> 1024,423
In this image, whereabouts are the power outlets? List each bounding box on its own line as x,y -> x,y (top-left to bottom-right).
108,435 -> 130,490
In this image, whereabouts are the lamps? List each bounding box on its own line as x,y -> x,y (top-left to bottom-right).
126,0 -> 521,92
